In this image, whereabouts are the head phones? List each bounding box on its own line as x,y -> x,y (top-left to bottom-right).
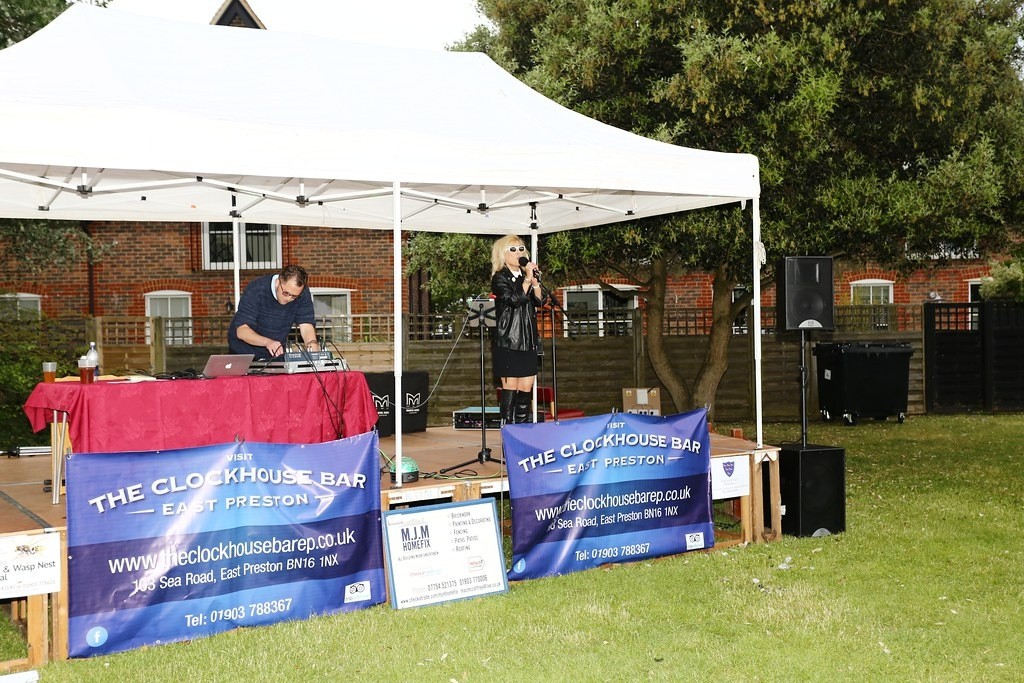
156,368 -> 196,380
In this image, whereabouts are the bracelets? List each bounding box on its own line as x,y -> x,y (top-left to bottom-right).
524,277 -> 532,284
532,283 -> 539,289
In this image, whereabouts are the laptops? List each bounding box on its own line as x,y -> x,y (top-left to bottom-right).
198,354 -> 256,378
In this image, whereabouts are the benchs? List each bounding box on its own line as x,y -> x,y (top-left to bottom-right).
497,386 -> 585,421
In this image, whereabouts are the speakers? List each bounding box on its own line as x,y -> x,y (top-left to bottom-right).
400,371 -> 429,434
775,255 -> 834,333
761,444 -> 847,538
362,371 -> 395,437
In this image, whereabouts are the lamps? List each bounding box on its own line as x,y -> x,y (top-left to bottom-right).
930,292 -> 944,303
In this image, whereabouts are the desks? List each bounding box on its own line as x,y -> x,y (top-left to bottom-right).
22,370 -> 379,504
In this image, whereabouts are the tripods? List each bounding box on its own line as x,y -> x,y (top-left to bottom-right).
0,446 -> 53,459
440,304 -> 506,474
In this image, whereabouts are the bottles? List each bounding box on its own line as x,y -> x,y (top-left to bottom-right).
87,342 -> 98,381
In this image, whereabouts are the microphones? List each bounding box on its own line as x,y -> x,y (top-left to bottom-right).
518,257 -> 539,275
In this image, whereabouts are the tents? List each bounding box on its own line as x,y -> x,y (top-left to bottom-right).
0,2 -> 762,485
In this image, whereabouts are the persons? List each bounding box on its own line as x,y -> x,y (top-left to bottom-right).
227,265 -> 319,369
490,235 -> 543,458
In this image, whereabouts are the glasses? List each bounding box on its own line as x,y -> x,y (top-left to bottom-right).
505,246 -> 525,253
279,278 -> 300,299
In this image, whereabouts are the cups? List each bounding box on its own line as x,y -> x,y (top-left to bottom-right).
42,362 -> 57,383
78,360 -> 95,384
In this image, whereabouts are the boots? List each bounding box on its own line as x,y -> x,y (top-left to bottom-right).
516,390 -> 531,424
500,388 -> 517,458
